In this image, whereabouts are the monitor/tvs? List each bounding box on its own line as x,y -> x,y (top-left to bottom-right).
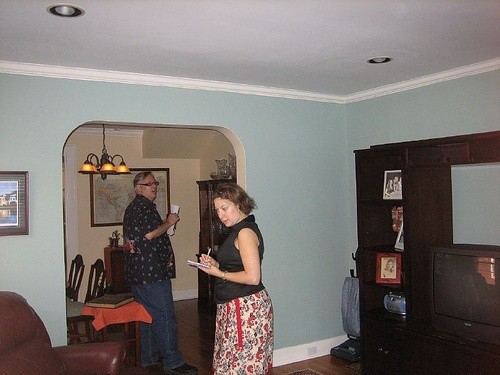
428,247 -> 500,346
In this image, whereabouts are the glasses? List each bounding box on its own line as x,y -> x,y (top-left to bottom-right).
136,181 -> 159,186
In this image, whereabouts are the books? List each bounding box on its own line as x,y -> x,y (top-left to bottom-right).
86,295 -> 135,309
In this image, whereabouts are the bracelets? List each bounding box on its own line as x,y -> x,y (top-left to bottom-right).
223,271 -> 228,283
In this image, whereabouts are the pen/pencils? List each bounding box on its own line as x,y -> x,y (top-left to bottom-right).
109,230 -> 121,239
204,248 -> 211,264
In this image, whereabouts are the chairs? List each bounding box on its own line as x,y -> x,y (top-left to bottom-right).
67,254 -> 107,343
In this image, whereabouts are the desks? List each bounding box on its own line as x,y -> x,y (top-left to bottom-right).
80,302 -> 153,368
67,296 -> 93,345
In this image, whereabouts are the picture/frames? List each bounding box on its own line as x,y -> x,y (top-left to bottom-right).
0,171 -> 29,236
90,168 -> 170,227
374,252 -> 401,284
382,169 -> 402,199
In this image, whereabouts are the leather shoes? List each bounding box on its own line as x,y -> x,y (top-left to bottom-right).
161,363 -> 198,375
143,363 -> 163,367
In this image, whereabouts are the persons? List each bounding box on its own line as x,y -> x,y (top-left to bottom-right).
383,176 -> 402,196
199,184 -> 274,375
382,260 -> 395,278
123,171 -> 198,374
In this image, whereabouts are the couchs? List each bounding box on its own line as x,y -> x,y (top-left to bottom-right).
0,291 -> 128,375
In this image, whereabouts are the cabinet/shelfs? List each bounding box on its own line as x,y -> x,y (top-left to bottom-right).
353,130 -> 500,375
104,247 -> 123,290
196,179 -> 237,314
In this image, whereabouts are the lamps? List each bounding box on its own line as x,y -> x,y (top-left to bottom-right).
77,124 -> 131,179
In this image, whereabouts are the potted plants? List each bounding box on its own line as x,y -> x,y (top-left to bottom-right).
108,230 -> 121,246
